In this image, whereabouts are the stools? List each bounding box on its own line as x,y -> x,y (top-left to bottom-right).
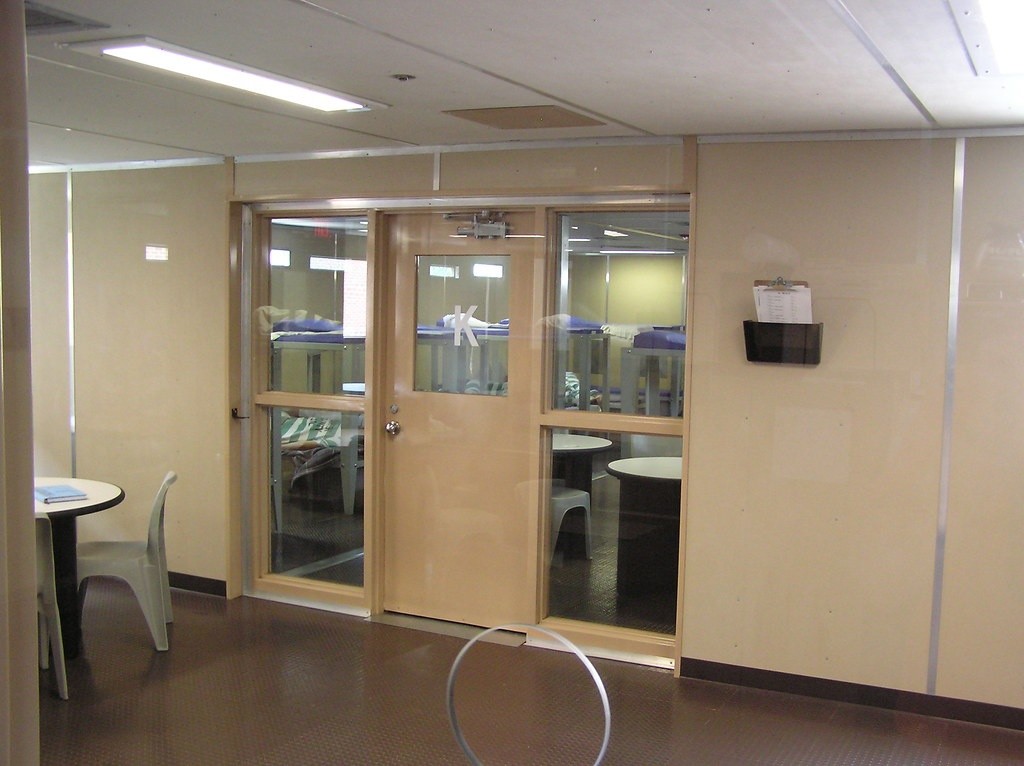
551,487 -> 592,562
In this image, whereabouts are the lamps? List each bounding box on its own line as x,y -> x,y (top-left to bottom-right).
61,36 -> 392,121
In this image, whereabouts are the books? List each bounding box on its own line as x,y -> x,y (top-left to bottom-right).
32,483 -> 87,504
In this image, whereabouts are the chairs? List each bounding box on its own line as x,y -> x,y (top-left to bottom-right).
77,471 -> 175,651
34,512 -> 69,700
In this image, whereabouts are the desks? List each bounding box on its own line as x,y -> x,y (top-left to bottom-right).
34,476 -> 124,657
607,457 -> 682,601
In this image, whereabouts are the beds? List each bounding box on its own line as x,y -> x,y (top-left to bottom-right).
270,312 -> 684,532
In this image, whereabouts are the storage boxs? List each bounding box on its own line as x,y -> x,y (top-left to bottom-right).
744,319 -> 822,364
